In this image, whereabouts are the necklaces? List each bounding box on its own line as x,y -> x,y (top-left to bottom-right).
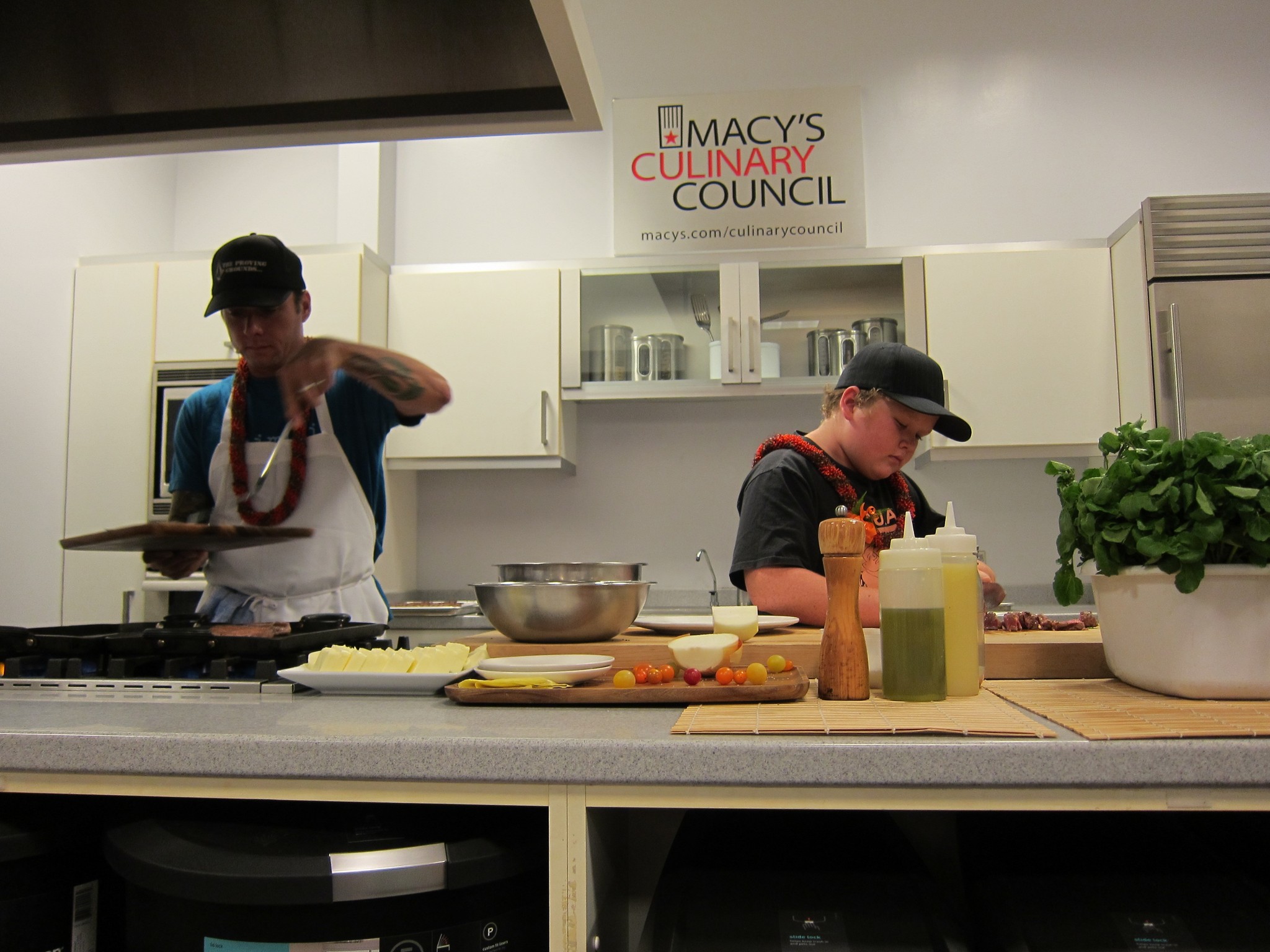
752,433 -> 915,549
228,357 -> 307,527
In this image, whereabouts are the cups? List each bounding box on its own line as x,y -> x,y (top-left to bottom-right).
760,342 -> 780,378
709,340 -> 721,380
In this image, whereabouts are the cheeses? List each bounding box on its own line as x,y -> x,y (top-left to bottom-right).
300,641 -> 489,673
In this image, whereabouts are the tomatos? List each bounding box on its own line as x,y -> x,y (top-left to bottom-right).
783,659 -> 793,671
746,662 -> 767,685
613,670 -> 636,688
767,654 -> 786,673
716,667 -> 734,685
734,669 -> 747,685
633,661 -> 680,684
684,668 -> 701,685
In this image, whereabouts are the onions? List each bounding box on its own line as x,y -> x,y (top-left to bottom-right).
712,605 -> 759,642
667,633 -> 742,676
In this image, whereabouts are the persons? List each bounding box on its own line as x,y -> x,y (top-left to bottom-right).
729,341 -> 995,630
142,232 -> 451,641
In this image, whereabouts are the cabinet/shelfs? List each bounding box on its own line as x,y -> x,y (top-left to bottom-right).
560,244 -> 925,402
61,247 -> 417,610
382,263 -> 577,474
923,241 -> 1124,457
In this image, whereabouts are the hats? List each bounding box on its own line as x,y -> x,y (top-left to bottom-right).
204,232 -> 306,316
835,343 -> 972,443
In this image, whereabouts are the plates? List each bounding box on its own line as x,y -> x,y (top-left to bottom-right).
474,654 -> 615,683
276,663 -> 474,696
443,664 -> 810,704
631,615 -> 800,633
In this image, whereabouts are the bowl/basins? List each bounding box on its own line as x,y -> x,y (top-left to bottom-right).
1077,558 -> 1270,701
465,580 -> 657,641
487,562 -> 649,580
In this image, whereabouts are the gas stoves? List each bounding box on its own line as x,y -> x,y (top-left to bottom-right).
0,612 -> 390,692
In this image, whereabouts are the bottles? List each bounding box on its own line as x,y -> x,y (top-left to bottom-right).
926,501 -> 984,696
877,511 -> 948,703
